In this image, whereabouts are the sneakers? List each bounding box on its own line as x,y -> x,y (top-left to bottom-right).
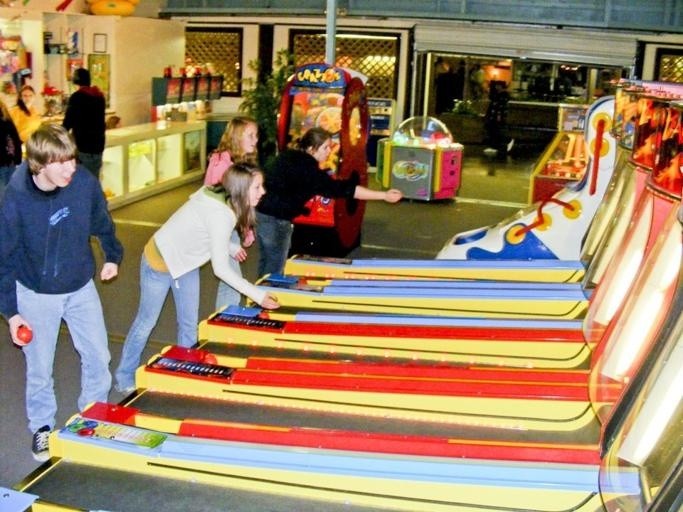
31,430 -> 52,462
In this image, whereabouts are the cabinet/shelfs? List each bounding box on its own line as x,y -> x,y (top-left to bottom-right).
21,9 -> 113,119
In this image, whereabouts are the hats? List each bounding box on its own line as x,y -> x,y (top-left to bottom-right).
72,67 -> 90,86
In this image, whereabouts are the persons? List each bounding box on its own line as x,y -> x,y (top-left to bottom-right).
113,162 -> 280,396
434,57 -> 485,115
0,85 -> 43,166
61,69 -> 106,178
480,80 -> 515,154
0,122 -> 123,462
252,127 -> 401,280
204,117 -> 258,312
